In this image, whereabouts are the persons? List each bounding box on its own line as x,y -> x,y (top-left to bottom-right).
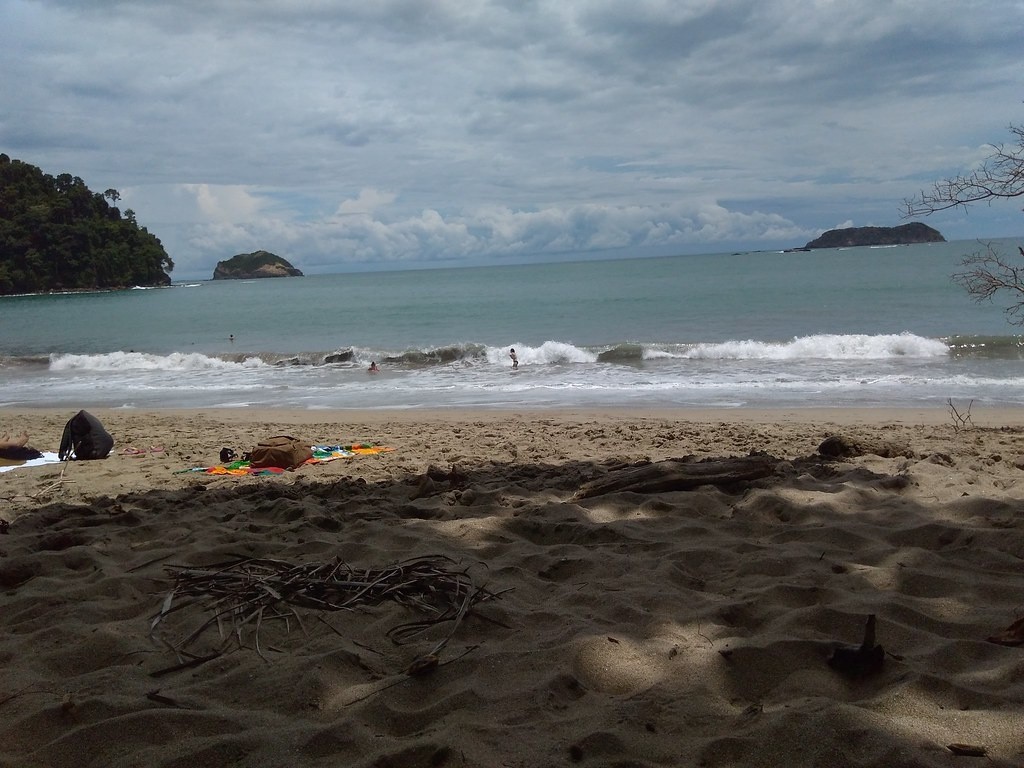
370,362 -> 379,371
509,349 -> 518,367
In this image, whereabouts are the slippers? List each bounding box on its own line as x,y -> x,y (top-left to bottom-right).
131,447 -> 146,452
117,449 -> 138,454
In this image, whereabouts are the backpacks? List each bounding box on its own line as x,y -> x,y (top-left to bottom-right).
58,409 -> 114,462
251,435 -> 314,469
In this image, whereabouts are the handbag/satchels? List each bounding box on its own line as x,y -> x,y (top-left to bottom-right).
219,447 -> 251,463
